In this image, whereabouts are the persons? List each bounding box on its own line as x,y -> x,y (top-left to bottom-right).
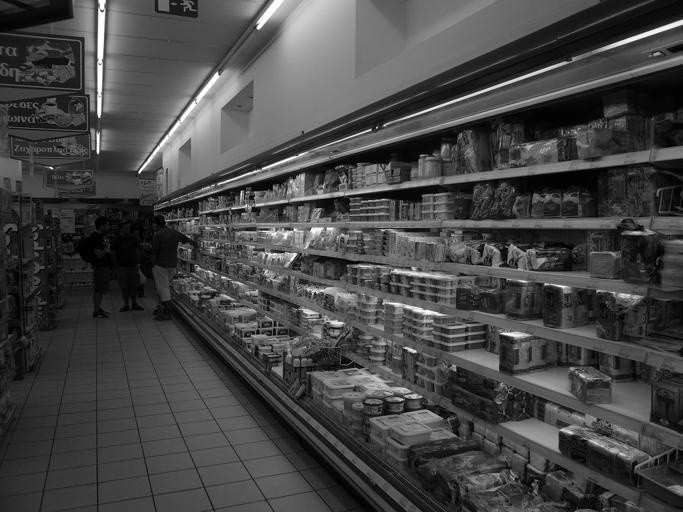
77,215 -> 197,321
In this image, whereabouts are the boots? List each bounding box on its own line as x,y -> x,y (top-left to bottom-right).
153,299 -> 172,320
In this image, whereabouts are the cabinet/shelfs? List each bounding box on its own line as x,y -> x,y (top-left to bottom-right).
0,206 -> 66,428
162,33 -> 682,512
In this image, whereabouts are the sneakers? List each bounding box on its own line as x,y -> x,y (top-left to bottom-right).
93,309 -> 112,319
132,305 -> 144,310
120,305 -> 129,312
